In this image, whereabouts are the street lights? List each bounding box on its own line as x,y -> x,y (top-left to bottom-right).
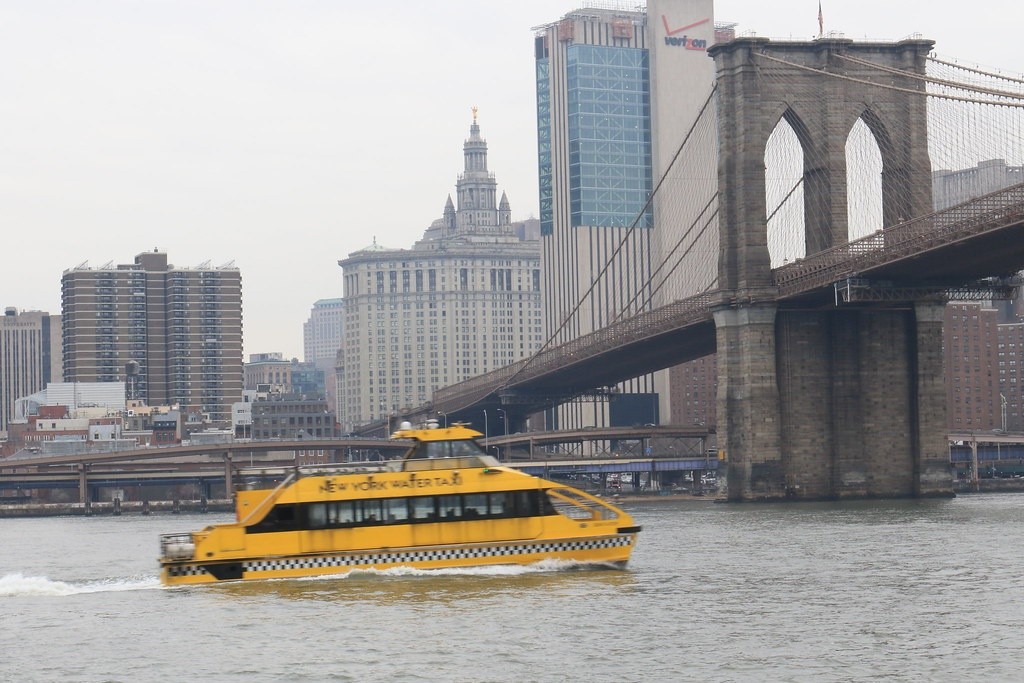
497,408 -> 507,435
499,416 -> 509,435
437,411 -> 447,428
492,446 -> 500,460
483,409 -> 488,452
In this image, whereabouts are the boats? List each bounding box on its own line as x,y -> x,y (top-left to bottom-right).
155,421 -> 647,588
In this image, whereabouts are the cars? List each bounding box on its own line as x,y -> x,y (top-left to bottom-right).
563,472 -> 647,496
